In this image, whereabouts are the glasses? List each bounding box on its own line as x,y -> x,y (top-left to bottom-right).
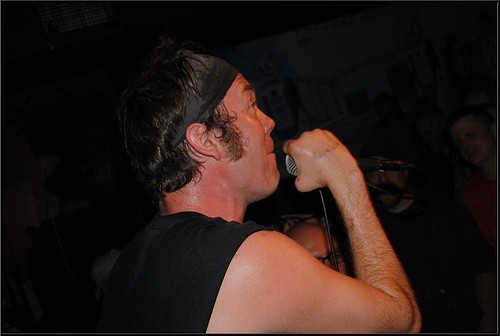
315,249 -> 347,264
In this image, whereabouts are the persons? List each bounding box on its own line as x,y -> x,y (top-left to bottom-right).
97,44 -> 424,334
276,87 -> 498,332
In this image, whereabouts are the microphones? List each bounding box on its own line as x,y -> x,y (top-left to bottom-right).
285,154 -> 410,176
